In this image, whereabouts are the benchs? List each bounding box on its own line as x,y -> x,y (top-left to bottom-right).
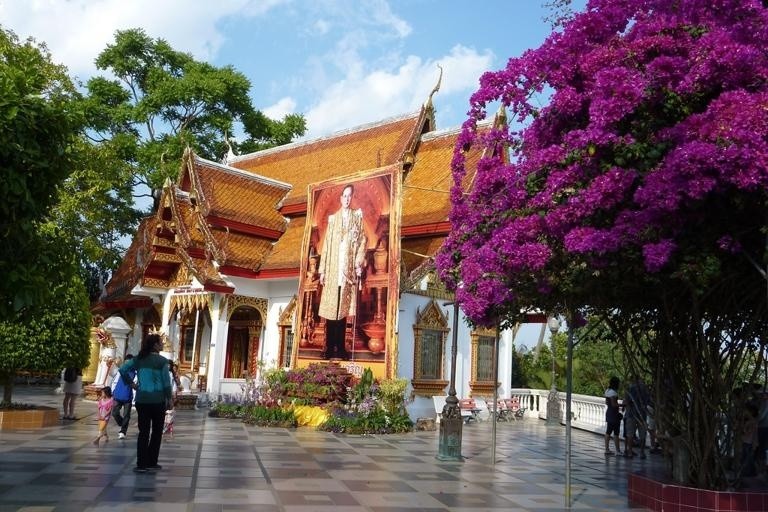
432,396 -> 528,424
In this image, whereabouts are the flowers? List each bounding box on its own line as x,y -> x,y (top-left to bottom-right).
435,6 -> 768,490
210,361 -> 415,434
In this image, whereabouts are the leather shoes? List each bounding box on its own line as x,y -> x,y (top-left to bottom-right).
323,349 -> 350,362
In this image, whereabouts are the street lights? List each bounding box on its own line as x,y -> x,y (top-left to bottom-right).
547,311 -> 562,426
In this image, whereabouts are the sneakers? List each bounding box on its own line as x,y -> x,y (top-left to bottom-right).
132,465 -> 162,473
92,432 -> 128,444
604,447 -> 659,459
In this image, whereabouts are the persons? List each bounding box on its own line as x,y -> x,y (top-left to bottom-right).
111,334 -> 184,473
605,375 -> 665,458
61,368 -> 82,419
319,185 -> 367,358
730,382 -> 768,477
93,387 -> 113,443
